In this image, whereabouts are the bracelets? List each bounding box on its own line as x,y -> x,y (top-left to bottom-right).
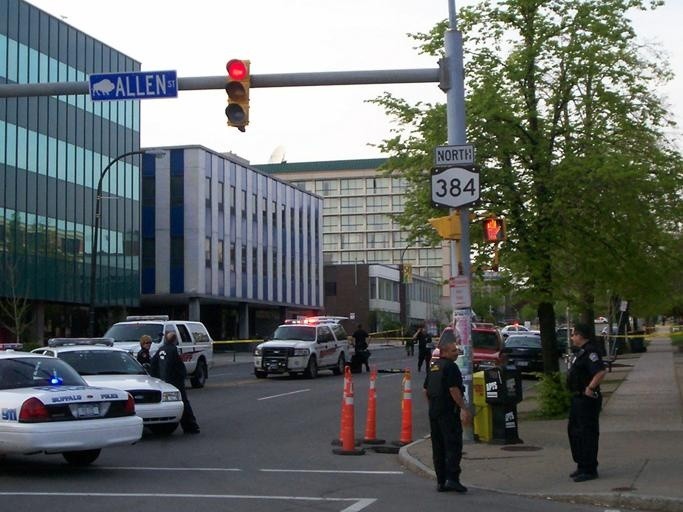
588,383 -> 596,391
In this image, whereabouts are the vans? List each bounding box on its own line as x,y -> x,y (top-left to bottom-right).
601,326 -> 621,334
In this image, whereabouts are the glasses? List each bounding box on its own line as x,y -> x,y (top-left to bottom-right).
570,333 -> 577,336
146,342 -> 152,344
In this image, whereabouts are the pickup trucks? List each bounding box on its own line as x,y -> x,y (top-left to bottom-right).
593,316 -> 608,324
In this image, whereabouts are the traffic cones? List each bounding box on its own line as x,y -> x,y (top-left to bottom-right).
331,364 -> 363,447
361,370 -> 386,445
333,381 -> 365,455
391,367 -> 419,447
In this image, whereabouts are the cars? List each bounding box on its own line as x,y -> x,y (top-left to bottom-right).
505,334 -> 543,377
502,324 -> 543,335
557,349 -> 558,352
0,351 -> 144,467
555,328 -> 574,350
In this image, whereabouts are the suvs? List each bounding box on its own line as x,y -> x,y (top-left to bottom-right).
255,318 -> 355,377
102,315 -> 215,389
429,323 -> 504,370
33,347 -> 186,438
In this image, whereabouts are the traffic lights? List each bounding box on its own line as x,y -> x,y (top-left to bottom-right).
226,58 -> 250,132
485,219 -> 503,242
429,210 -> 461,240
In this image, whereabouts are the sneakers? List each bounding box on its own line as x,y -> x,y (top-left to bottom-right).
438,484 -> 447,492
574,473 -> 598,482
445,480 -> 467,492
570,470 -> 581,477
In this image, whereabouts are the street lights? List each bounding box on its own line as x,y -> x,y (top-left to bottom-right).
86,148 -> 168,334
398,239 -> 431,326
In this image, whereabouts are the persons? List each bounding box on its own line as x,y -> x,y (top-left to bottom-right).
136,335 -> 152,366
422,339 -> 471,492
151,332 -> 200,434
563,322 -> 608,482
402,328 -> 417,356
412,326 -> 432,373
351,323 -> 370,373
